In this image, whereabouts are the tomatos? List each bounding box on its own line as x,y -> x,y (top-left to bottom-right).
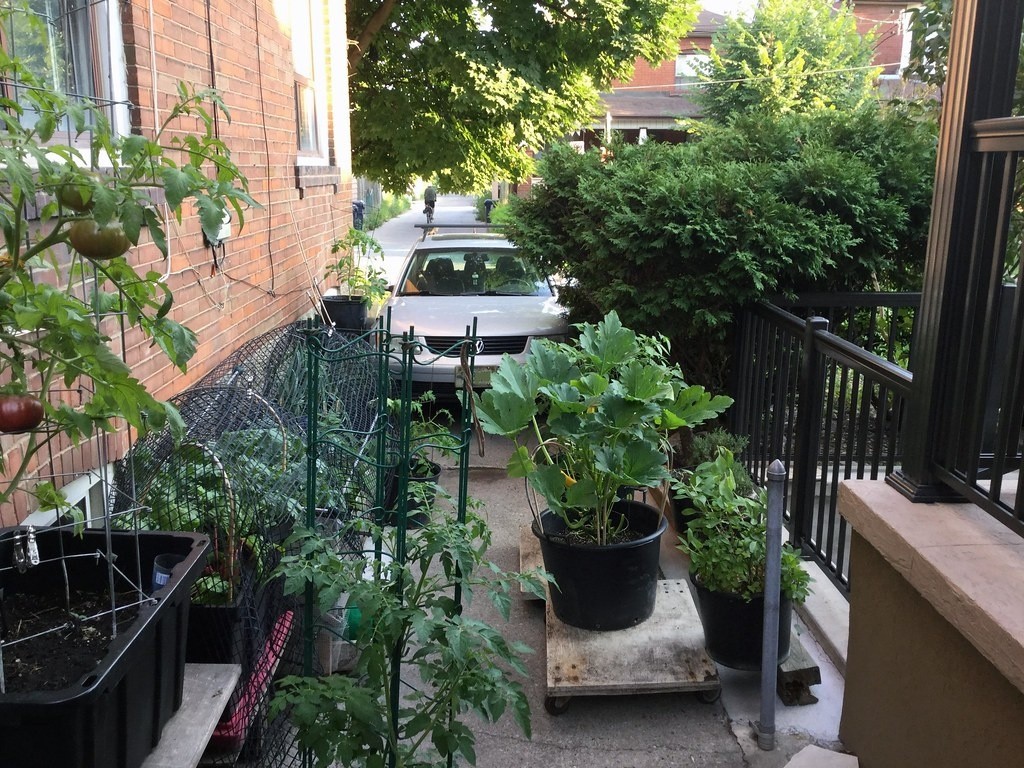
55,169 -> 106,211
0,391 -> 44,434
71,212 -> 131,259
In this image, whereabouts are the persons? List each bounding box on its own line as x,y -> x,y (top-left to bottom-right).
423,185 -> 436,219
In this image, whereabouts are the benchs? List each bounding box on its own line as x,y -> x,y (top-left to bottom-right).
422,269 -> 540,294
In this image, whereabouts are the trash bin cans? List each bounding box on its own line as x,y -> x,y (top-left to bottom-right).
353,200 -> 364,232
483,199 -> 496,223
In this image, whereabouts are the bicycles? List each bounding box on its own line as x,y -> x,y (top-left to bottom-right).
426,203 -> 433,223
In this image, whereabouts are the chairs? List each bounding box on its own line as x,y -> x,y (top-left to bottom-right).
427,258 -> 466,294
491,256 -> 524,292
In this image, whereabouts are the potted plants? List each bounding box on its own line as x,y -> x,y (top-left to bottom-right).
662,428 -> 754,538
456,309 -> 734,631
366,389 -> 460,530
667,446 -> 816,672
319,224 -> 389,335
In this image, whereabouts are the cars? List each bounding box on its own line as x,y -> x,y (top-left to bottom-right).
370,224 -> 571,404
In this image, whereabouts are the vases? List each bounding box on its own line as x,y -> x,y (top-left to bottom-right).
0,526 -> 210,768
